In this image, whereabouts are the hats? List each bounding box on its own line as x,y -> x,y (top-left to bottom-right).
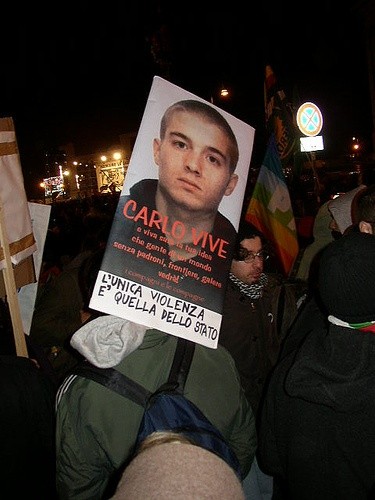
109,442 -> 244,500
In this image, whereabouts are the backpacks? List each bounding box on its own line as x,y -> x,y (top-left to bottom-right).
255,296 -> 375,480
68,337 -> 243,487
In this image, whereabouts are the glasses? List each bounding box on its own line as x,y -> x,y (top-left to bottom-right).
242,250 -> 269,263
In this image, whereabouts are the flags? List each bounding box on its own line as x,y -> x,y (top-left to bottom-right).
264,65 -> 311,185
0,117 -> 38,297
240,132 -> 300,280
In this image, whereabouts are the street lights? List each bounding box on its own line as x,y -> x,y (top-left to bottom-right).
209,87 -> 229,104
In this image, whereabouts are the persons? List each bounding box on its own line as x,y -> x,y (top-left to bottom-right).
99,100 -> 239,315
0,192 -> 121,499
274,186 -> 374,362
55,245 -> 258,500
256,232 -> 375,500
219,219 -> 300,500
